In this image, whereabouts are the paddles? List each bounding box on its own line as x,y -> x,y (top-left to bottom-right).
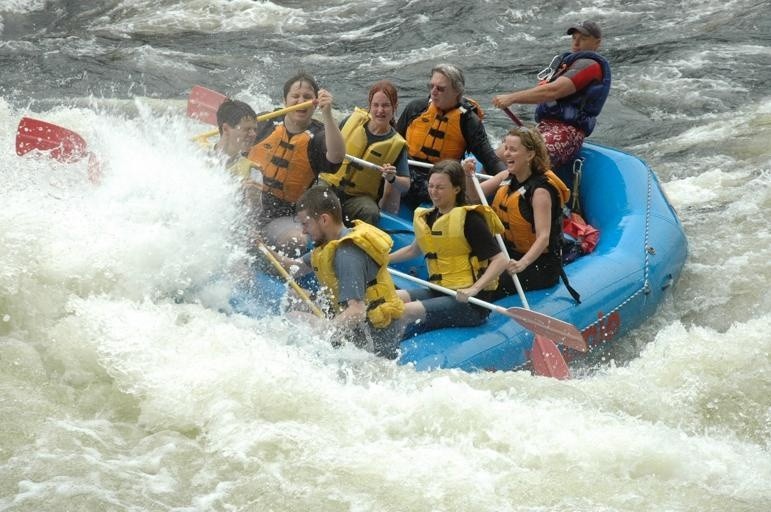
188,83 -> 386,172
468,159 -> 569,380
388,264 -> 586,352
16,117 -> 88,164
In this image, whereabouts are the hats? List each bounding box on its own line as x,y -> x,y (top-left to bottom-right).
567,20 -> 600,38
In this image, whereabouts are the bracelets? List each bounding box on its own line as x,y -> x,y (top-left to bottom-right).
384,176 -> 396,186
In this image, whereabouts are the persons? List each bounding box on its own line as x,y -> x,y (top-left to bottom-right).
240,77 -> 349,224
377,157 -> 512,341
314,80 -> 411,226
188,97 -> 267,229
246,184 -> 407,369
460,125 -> 563,304
395,62 -> 511,214
481,20 -> 610,186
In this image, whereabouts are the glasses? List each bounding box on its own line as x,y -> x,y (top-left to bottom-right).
427,83 -> 448,93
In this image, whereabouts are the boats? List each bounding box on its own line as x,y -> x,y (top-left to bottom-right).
150,117 -> 690,389
